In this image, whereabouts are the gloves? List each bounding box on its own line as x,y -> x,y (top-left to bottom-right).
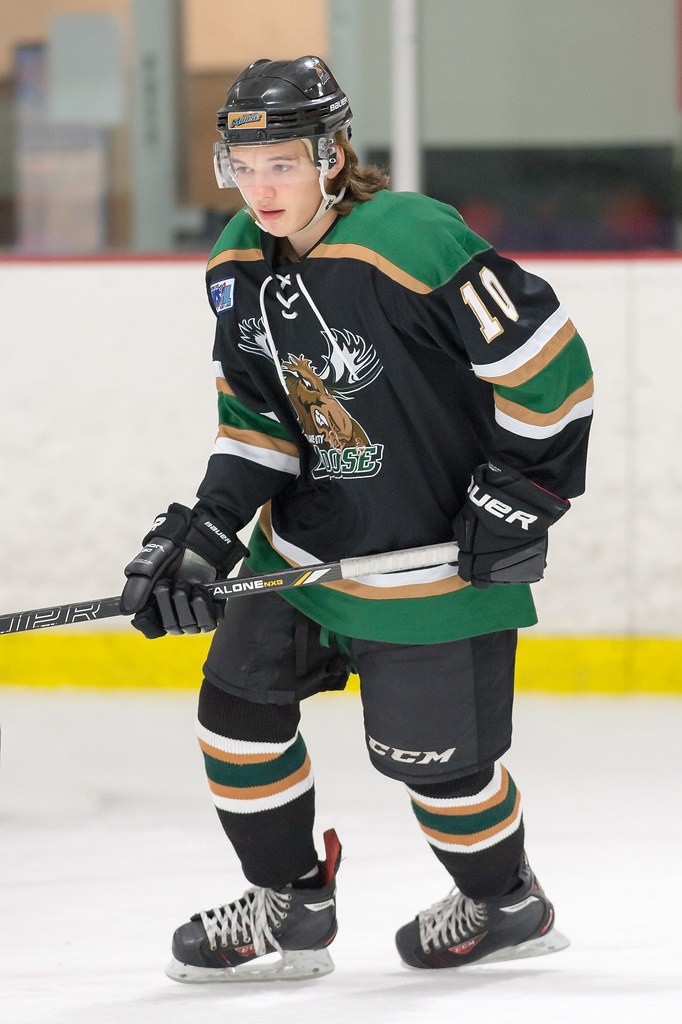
119,502 -> 250,639
454,461 -> 571,589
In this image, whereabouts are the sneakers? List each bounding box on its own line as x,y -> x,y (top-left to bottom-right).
395,851 -> 555,970
170,828 -> 341,968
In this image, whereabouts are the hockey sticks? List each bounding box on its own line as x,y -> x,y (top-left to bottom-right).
0,541 -> 457,636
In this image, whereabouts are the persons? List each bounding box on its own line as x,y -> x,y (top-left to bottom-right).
120,52 -> 595,984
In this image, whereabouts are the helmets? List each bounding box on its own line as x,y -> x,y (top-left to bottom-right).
214,55 -> 356,172
